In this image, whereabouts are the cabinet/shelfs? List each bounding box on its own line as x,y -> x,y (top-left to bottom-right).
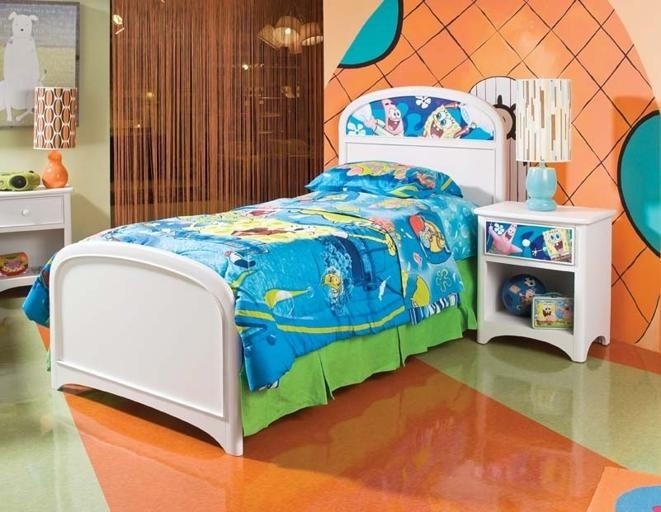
0,187 -> 74,293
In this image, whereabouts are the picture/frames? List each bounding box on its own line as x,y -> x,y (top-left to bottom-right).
1,1 -> 79,129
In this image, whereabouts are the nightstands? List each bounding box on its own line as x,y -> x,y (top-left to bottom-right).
472,201 -> 616,363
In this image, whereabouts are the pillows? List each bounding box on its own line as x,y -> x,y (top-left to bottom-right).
304,161 -> 462,199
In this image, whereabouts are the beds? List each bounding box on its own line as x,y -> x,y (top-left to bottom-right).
49,86 -> 508,458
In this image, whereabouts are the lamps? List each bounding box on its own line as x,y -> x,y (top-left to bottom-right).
516,78 -> 570,211
259,1 -> 323,57
33,86 -> 79,188
